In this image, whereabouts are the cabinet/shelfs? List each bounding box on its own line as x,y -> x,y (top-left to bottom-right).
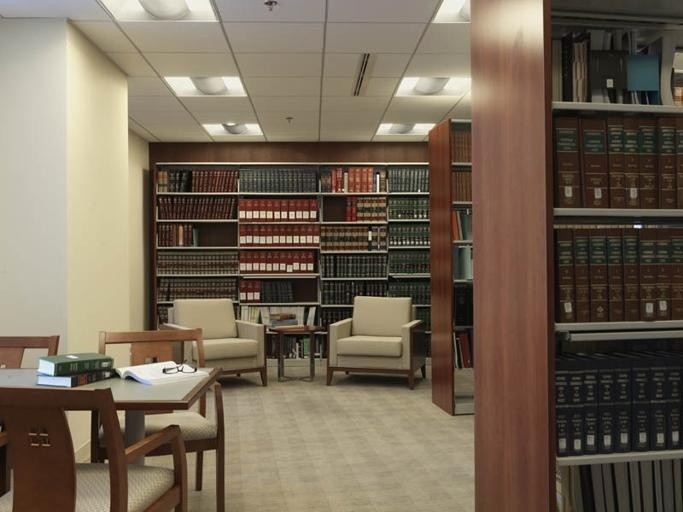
469,0 -> 682,510
149,143 -> 433,362
427,118 -> 473,417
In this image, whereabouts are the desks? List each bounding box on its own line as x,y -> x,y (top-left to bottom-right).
268,325 -> 327,382
2,362 -> 224,511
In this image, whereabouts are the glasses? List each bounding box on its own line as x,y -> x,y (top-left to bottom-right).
163,360 -> 197,374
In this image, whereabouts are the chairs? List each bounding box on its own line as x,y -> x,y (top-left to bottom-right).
0,333 -> 61,497
90,328 -> 224,512
327,295 -> 428,392
0,385 -> 192,512
165,297 -> 268,388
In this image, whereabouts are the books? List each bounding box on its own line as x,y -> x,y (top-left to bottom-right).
36,353 -> 210,388
553,113 -> 683,209
156,166 -> 431,358
554,228 -> 682,322
550,29 -> 682,105
450,128 -> 474,369
553,348 -> 683,456
554,459 -> 683,512
